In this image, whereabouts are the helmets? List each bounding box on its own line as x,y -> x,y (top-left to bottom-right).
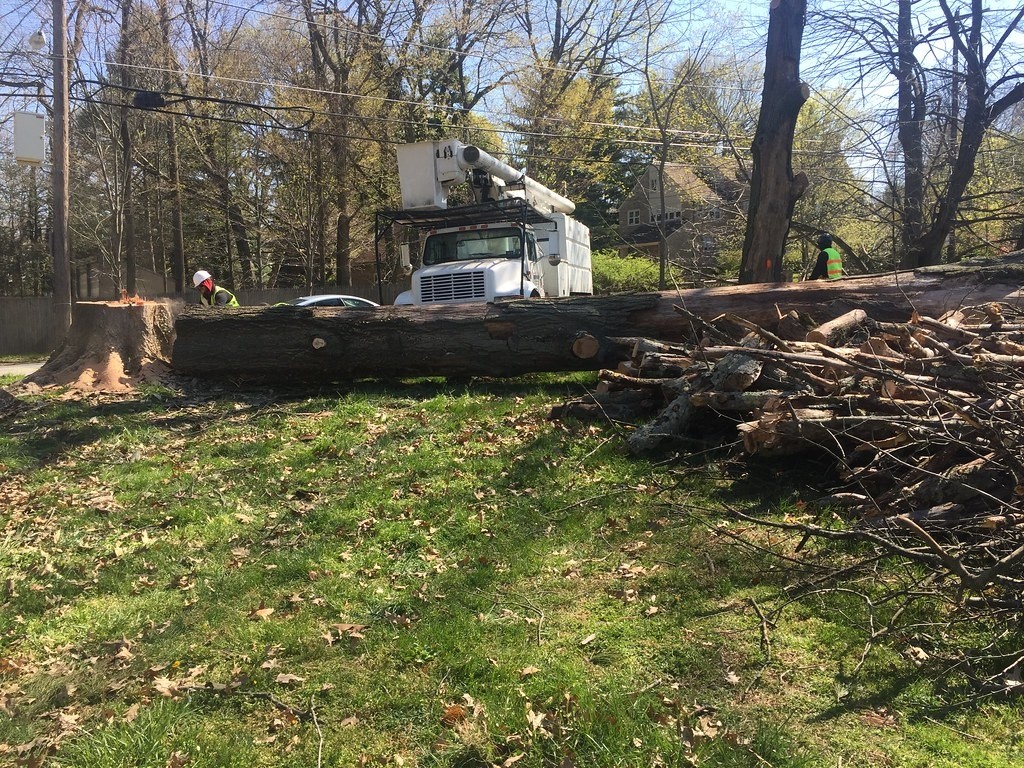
193,270 -> 212,290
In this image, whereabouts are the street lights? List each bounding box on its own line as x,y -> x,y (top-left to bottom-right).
29,17 -> 71,343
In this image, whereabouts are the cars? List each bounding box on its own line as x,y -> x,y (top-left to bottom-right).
286,294 -> 380,307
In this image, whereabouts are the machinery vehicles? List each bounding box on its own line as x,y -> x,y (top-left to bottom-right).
394,137 -> 593,305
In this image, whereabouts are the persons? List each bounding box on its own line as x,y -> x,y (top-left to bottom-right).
193,270 -> 240,306
812,233 -> 842,280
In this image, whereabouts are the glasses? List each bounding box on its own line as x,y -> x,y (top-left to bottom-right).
197,285 -> 204,289
